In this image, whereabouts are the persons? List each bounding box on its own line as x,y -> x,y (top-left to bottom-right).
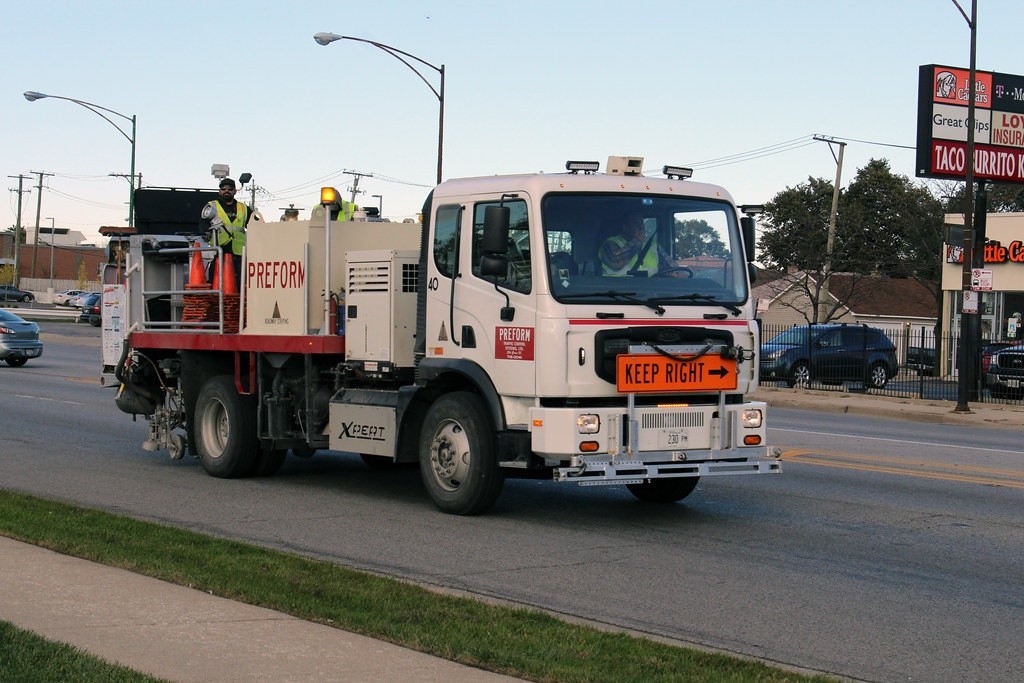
207,178 -> 258,292
312,200 -> 365,222
597,208 -> 689,278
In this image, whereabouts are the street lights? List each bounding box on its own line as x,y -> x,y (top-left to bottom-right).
24,90 -> 139,228
45,217 -> 54,288
311,30 -> 447,192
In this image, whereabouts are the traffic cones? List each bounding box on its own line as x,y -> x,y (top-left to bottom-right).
180,241 -> 246,334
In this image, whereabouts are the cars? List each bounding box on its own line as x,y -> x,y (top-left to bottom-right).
985,344 -> 1024,401
0,309 -> 41,368
53,287 -> 86,307
67,293 -> 93,308
78,292 -> 102,326
980,340 -> 1024,378
907,346 -> 936,376
0,285 -> 35,303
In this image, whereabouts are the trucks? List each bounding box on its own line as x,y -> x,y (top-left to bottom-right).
96,158 -> 782,519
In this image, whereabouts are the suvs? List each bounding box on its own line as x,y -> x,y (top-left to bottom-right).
759,323 -> 900,390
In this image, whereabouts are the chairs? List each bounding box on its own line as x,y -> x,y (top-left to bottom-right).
593,220 -> 624,276
